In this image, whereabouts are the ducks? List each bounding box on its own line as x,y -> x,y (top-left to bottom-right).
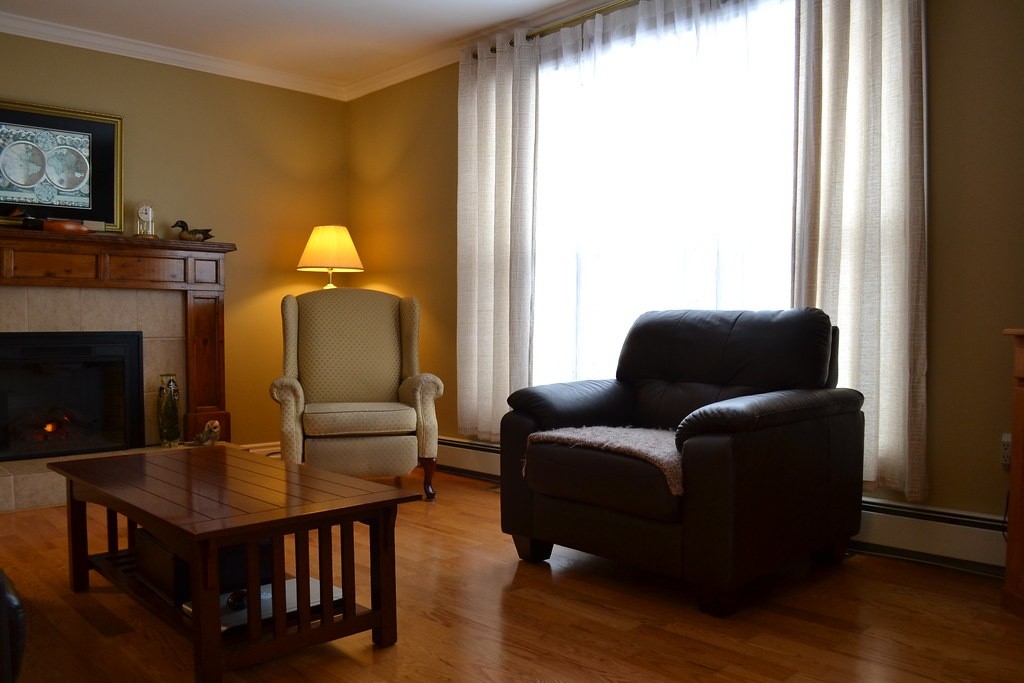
171,220 -> 215,241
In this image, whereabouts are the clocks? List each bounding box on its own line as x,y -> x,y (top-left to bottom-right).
138,206 -> 155,221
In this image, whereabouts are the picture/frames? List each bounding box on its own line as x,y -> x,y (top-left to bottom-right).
1,97 -> 124,234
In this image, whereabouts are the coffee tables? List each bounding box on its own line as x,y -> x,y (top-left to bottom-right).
48,446 -> 425,683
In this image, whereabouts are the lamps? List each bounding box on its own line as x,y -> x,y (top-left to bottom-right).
297,225 -> 366,290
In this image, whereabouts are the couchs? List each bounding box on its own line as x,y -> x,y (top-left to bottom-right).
271,286 -> 444,500
497,308 -> 864,617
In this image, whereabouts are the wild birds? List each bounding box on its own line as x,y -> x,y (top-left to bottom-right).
178,420 -> 221,447
